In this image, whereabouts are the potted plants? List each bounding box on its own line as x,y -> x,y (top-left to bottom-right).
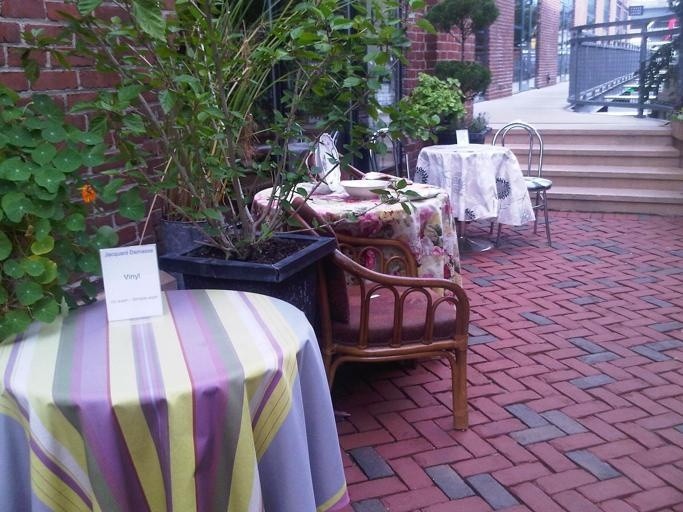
0,2 -> 473,343
419,0 -> 503,146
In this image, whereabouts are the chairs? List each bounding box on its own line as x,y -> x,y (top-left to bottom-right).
278,198 -> 472,434
488,118 -> 555,247
369,126 -> 403,184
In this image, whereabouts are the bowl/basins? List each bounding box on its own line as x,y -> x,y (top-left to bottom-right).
337,178 -> 390,200
314,132 -> 340,192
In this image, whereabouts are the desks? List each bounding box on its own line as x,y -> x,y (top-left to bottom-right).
3,289 -> 354,508
251,179 -> 464,326
413,144 -> 538,246
